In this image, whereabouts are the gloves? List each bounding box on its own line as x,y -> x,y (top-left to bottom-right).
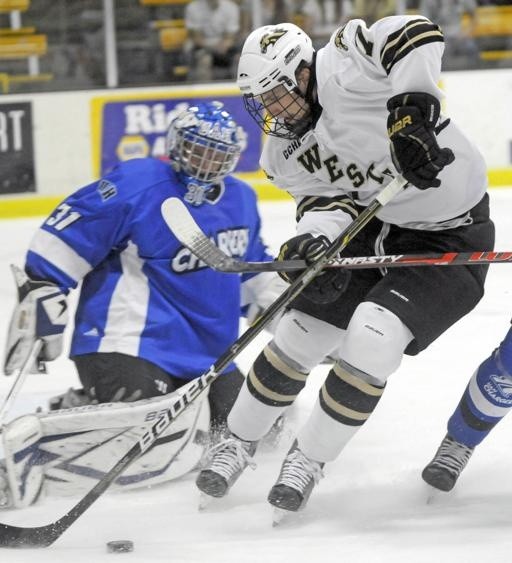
381,91 -> 456,191
276,233 -> 353,306
1,261 -> 68,378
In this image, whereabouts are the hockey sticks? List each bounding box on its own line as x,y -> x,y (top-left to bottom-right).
162,196 -> 512,274
0,173 -> 410,548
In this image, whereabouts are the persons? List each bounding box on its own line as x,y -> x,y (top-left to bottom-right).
178,0 -> 488,82
4,102 -> 291,487
198,15 -> 493,506
423,324 -> 512,495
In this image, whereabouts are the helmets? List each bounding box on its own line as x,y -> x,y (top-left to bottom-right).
235,22 -> 316,100
164,99 -> 249,183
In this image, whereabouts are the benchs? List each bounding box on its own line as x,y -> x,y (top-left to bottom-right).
141,1 -> 512,76
0,1 -> 54,91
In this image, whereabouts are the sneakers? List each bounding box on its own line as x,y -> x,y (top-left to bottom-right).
421,433 -> 475,493
267,439 -> 326,513
194,431 -> 260,499
